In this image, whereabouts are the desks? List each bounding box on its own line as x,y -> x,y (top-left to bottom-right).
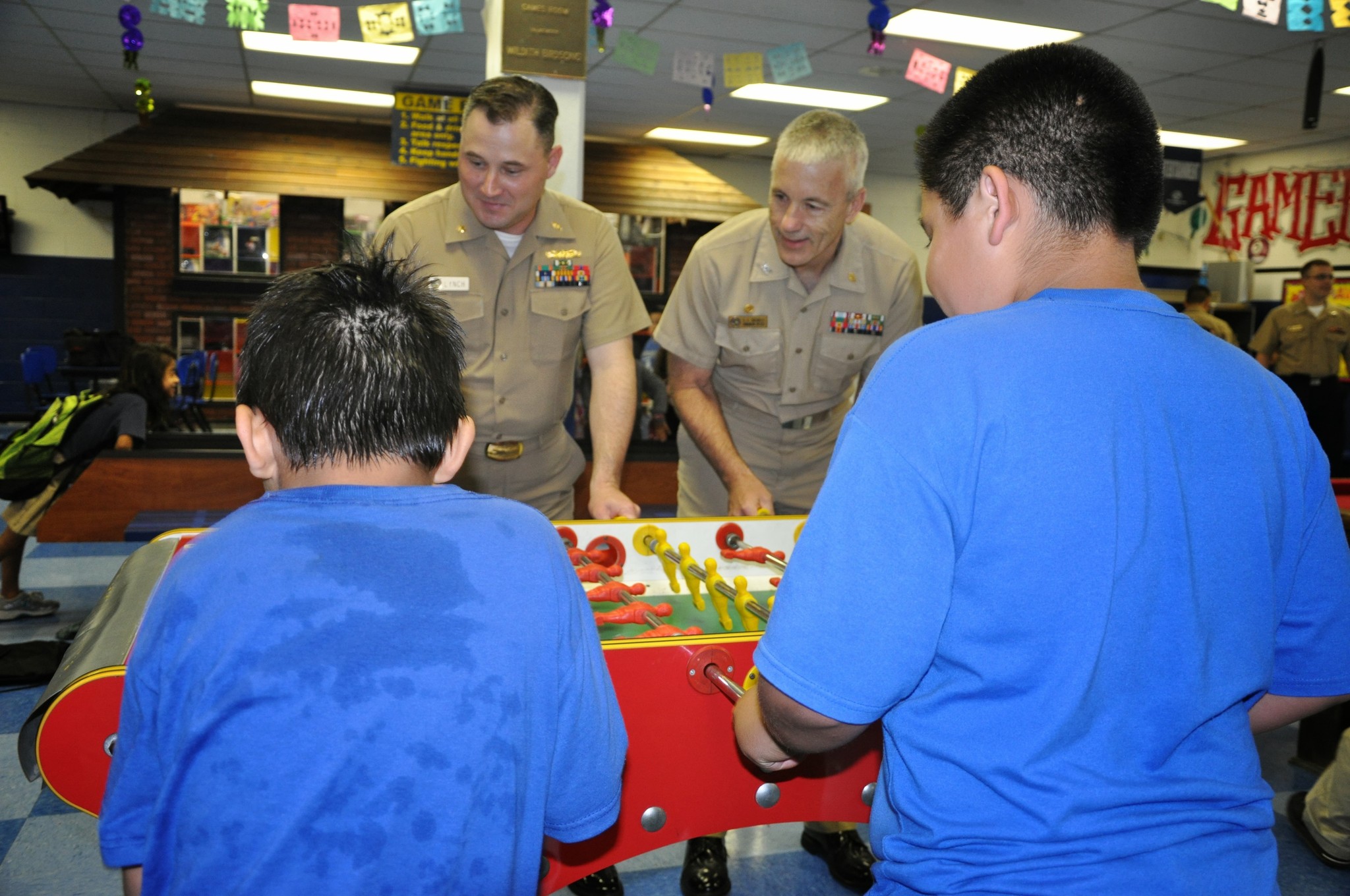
59,328 -> 133,399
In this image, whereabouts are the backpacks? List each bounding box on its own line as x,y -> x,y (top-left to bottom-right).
0,387 -> 111,501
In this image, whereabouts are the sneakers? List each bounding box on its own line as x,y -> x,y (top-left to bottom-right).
0,590 -> 61,621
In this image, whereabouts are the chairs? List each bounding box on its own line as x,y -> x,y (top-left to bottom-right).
18,343 -> 76,422
167,349 -> 219,435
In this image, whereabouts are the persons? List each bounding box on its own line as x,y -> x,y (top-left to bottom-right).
652,107 -> 925,896
576,353 -> 672,443
1284,713 -> 1350,874
0,341 -> 181,623
363,73 -> 659,895
1246,258 -> 1349,480
1180,283 -> 1243,352
99,227 -> 632,896
730,43 -> 1349,896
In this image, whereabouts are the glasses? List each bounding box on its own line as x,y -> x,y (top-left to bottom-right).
1306,273 -> 1334,281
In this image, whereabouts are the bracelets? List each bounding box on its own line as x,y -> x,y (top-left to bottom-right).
648,417 -> 666,427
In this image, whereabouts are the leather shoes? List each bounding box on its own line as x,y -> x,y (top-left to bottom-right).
568,865 -> 624,896
800,825 -> 877,895
680,836 -> 731,896
